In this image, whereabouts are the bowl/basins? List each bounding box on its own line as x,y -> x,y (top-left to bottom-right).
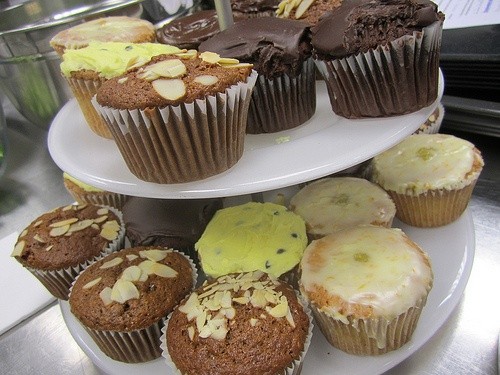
0,104 -> 8,187
0,0 -> 212,131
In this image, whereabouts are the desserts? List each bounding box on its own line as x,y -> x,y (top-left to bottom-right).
49,0 -> 445,184
8,96 -> 485,375
